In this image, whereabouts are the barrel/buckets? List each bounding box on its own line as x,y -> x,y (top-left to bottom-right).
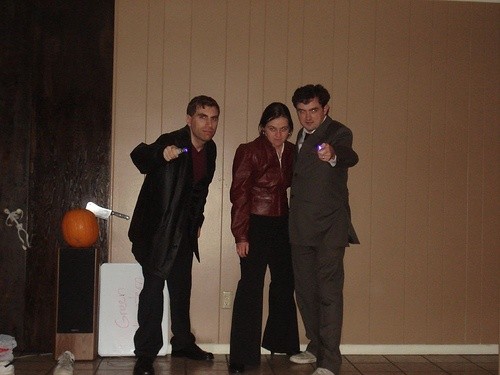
0,359 -> 15,375
0,334 -> 17,361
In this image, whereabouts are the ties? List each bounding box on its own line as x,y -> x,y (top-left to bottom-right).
304,133 -> 310,141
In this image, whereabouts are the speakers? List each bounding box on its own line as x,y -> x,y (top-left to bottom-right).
56,247 -> 99,361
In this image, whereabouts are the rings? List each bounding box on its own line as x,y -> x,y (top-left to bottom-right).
322,156 -> 324,159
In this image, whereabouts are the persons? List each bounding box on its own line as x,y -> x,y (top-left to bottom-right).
128,95 -> 220,375
228,101 -> 296,375
289,83 -> 361,375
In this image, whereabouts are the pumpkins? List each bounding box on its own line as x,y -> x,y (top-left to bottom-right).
61,207 -> 98,247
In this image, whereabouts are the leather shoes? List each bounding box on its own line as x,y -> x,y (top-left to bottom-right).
170,343 -> 212,361
228,360 -> 246,374
270,349 -> 303,357
133,356 -> 155,375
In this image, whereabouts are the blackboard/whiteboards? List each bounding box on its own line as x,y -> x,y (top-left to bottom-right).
97,263 -> 175,357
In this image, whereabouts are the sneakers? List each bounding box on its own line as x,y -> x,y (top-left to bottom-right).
289,350 -> 317,364
311,366 -> 336,375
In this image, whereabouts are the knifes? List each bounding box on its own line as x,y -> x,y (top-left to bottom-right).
85,202 -> 130,221
